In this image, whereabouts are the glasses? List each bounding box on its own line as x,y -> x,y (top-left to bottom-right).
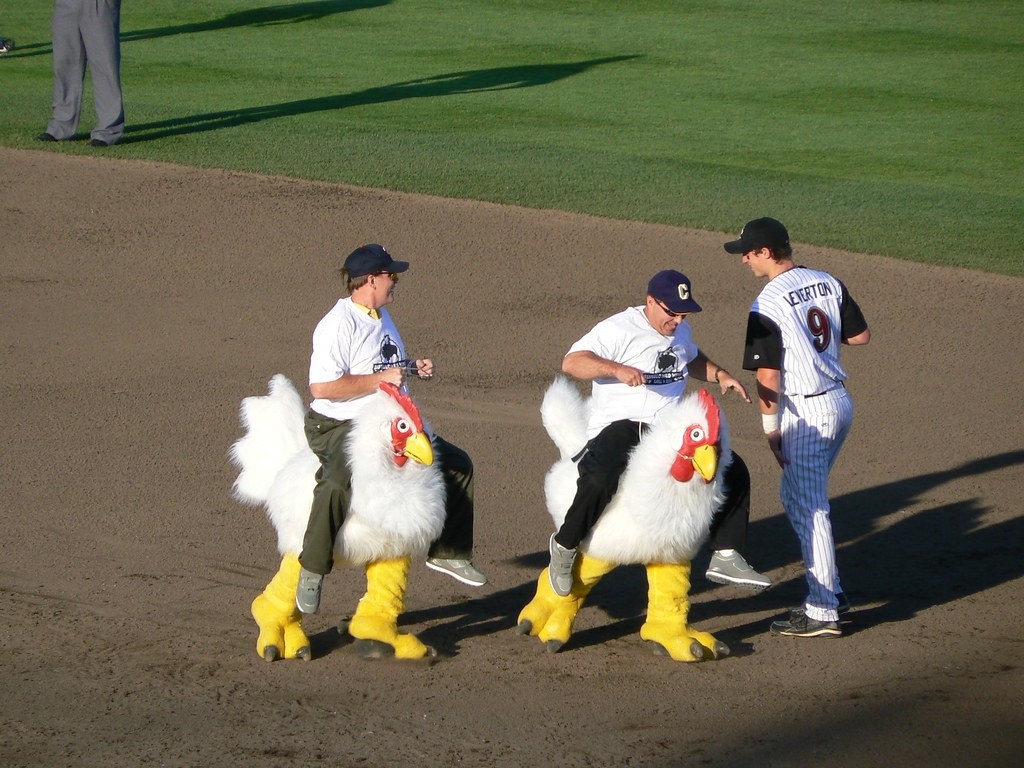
369,271 -> 397,281
654,297 -> 686,319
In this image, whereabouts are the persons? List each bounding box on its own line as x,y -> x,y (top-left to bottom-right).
294,244 -> 489,614
723,218 -> 873,641
32,0 -> 125,146
548,270 -> 753,596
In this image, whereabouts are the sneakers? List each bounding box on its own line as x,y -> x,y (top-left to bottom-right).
548,531 -> 578,595
791,593 -> 852,617
769,616 -> 842,637
425,557 -> 487,586
705,549 -> 772,591
296,564 -> 323,613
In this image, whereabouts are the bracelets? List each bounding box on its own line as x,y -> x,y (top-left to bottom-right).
761,413 -> 779,433
406,361 -> 414,375
715,368 -> 729,382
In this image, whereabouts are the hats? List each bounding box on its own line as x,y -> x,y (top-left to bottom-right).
346,243 -> 410,278
648,270 -> 702,312
724,218 -> 789,254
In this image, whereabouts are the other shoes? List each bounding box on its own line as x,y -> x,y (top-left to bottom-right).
0,38 -> 13,53
33,132 -> 56,143
87,139 -> 107,148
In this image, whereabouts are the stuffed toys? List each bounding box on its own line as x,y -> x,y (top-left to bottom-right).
224,371 -> 446,661
514,376 -> 733,663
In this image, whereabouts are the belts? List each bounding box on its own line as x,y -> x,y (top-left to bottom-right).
804,380 -> 845,399
